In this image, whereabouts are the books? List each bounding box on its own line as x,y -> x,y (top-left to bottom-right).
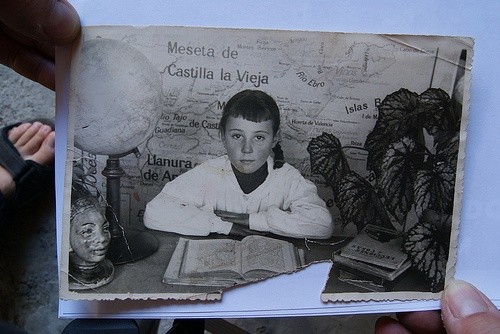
162,235 -> 304,287
331,223 -> 414,291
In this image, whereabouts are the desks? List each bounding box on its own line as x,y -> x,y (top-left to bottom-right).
70,236 -> 445,293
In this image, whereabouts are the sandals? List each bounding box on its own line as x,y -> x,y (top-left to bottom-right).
0,118 -> 55,219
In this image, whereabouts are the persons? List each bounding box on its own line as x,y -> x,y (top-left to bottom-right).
142,91 -> 334,238
71,184 -> 112,266
0,0 -> 500,334
